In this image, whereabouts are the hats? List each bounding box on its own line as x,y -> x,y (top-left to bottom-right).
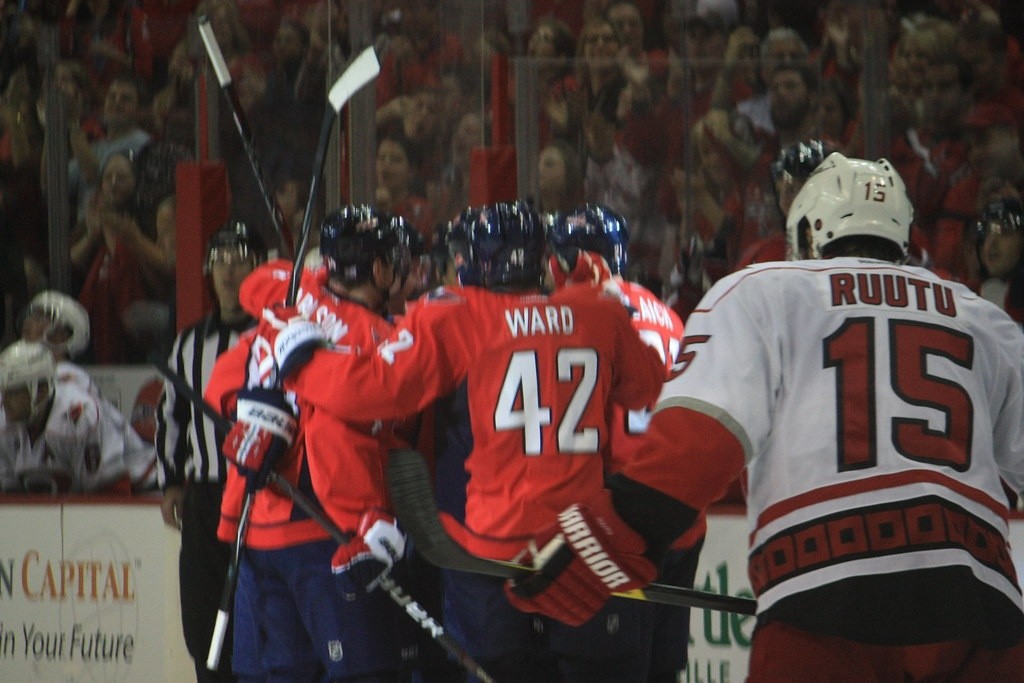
958,101 -> 1022,129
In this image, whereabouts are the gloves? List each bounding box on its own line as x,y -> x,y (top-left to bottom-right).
503,487 -> 658,628
222,387 -> 299,494
266,304 -> 326,387
331,509 -> 409,608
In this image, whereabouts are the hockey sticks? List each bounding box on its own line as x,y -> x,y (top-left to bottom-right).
195,15 -> 295,260
202,41 -> 382,673
384,447 -> 757,618
146,348 -> 497,683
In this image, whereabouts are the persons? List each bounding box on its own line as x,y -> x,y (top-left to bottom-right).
0,0 -> 1024,368
0,290 -> 90,491
0,339 -> 181,497
153,219 -> 267,683
197,201 -> 706,682
500,151 -> 1024,683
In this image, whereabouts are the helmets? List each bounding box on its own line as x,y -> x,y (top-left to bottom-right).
385,215 -> 425,276
320,203 -> 397,289
29,290 -> 90,360
431,222 -> 448,258
205,220 -> 268,301
468,201 -> 545,285
550,200 -> 626,277
782,139 -> 828,181
447,205 -> 481,286
0,338 -> 55,425
130,382 -> 164,445
976,199 -> 1024,236
786,152 -> 913,262
